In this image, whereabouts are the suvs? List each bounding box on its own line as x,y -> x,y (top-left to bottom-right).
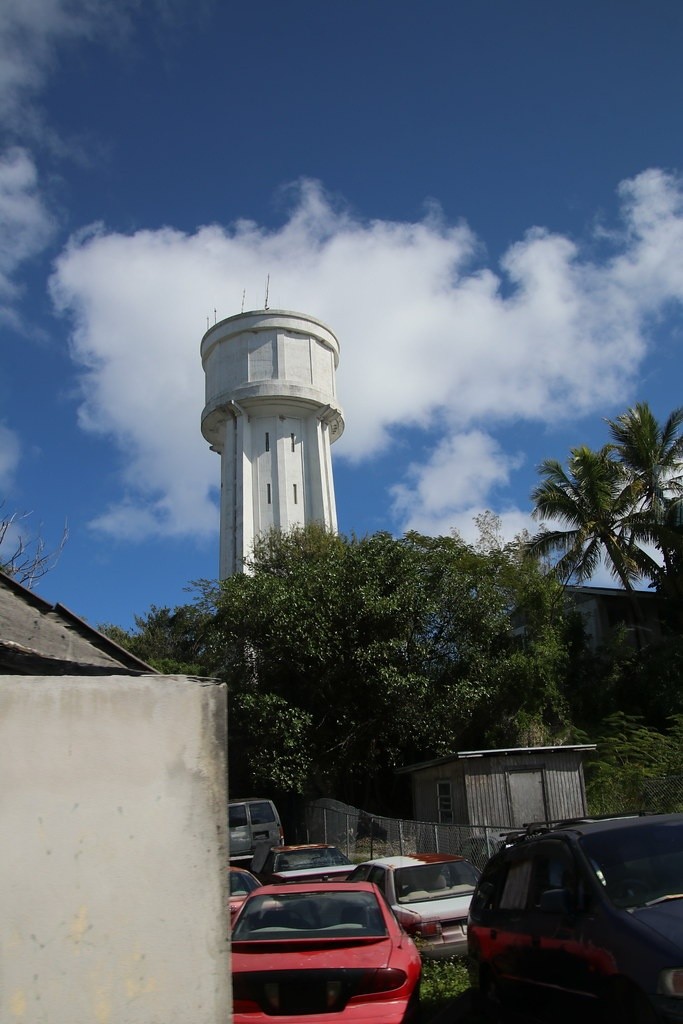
464,811 -> 683,1024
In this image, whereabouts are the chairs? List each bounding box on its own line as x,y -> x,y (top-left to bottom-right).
432,875 -> 447,889
340,907 -> 370,925
279,860 -> 289,866
311,857 -> 328,863
561,876 -> 592,898
259,910 -> 303,929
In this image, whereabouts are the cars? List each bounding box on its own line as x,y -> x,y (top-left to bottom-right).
340,853 -> 489,962
229,797 -> 285,864
231,880 -> 423,1024
255,843 -> 361,889
229,865 -> 284,931
